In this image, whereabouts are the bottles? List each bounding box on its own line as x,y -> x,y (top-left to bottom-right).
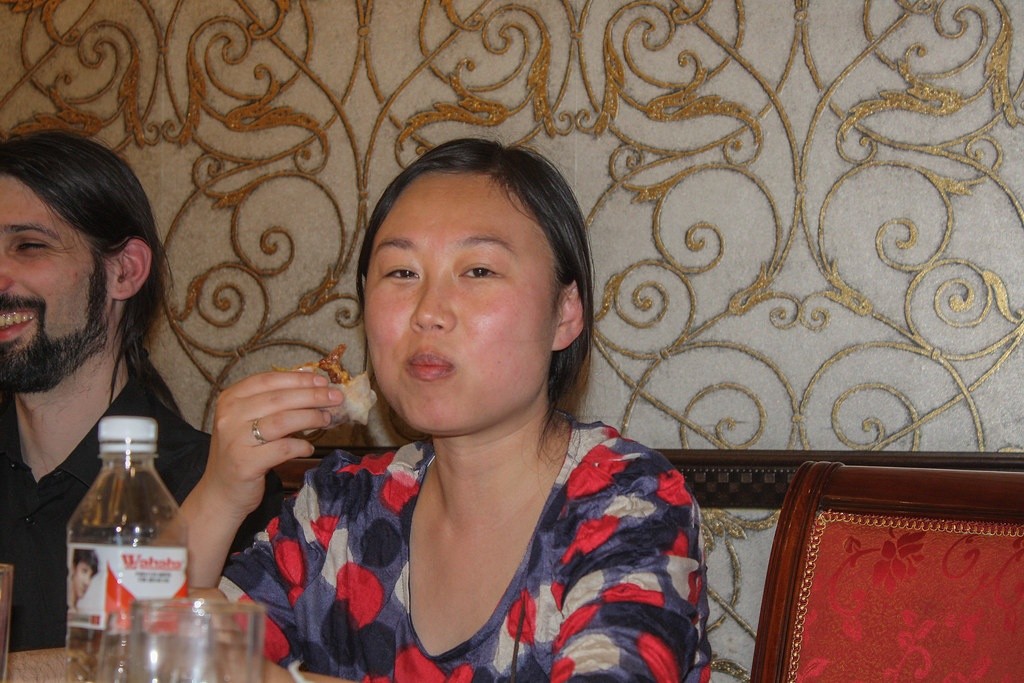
65,414 -> 190,683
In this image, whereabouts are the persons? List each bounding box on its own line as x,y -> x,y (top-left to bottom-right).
66,549 -> 99,611
0,131 -> 284,656
155,139 -> 712,683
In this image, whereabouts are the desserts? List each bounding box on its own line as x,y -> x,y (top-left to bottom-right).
271,343 -> 375,428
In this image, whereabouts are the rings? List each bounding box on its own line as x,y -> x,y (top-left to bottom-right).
252,418 -> 269,444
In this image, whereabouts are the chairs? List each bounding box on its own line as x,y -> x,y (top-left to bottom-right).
750,462 -> 1024,681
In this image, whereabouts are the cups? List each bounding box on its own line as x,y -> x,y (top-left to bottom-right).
0,563 -> 15,682
128,596 -> 268,683
